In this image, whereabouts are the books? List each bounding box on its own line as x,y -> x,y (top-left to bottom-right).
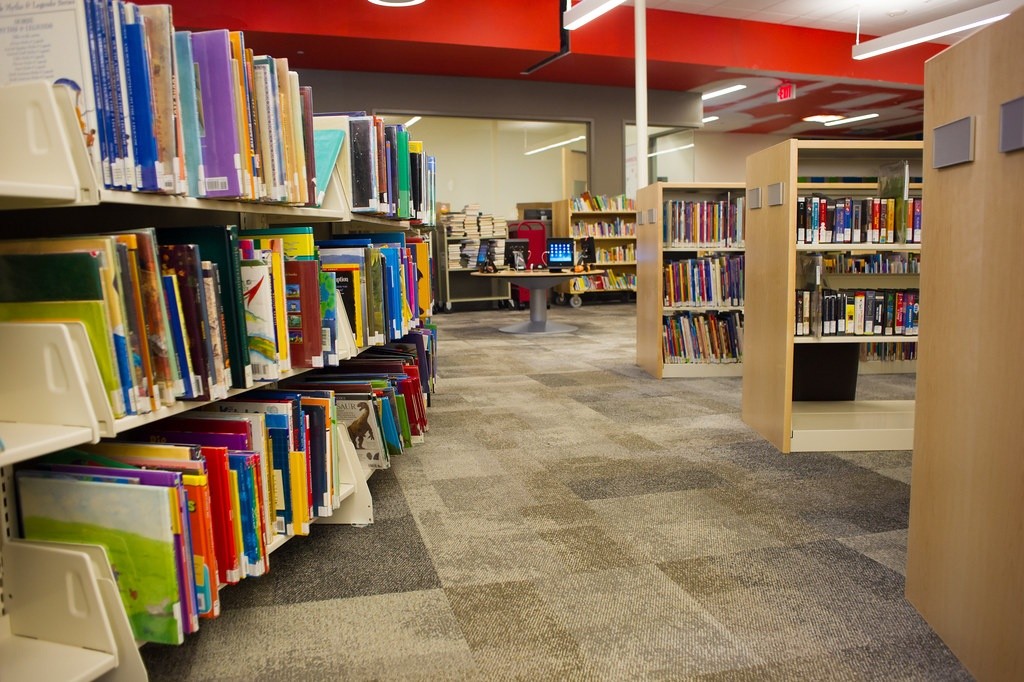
0,0 -> 439,647
796,159 -> 923,362
663,197 -> 745,364
571,191 -> 637,292
441,205 -> 507,268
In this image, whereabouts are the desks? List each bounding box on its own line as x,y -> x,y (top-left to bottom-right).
472,269 -> 605,335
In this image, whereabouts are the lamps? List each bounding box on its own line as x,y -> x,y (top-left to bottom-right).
562,0 -> 631,30
851,0 -> 1024,60
524,130 -> 586,155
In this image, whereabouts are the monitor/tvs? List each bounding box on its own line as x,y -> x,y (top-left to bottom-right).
504,239 -> 529,270
546,237 -> 575,273
577,237 -> 596,272
476,239 -> 498,273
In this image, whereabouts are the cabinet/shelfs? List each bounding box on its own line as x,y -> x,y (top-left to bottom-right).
1,7 -> 1024,682
